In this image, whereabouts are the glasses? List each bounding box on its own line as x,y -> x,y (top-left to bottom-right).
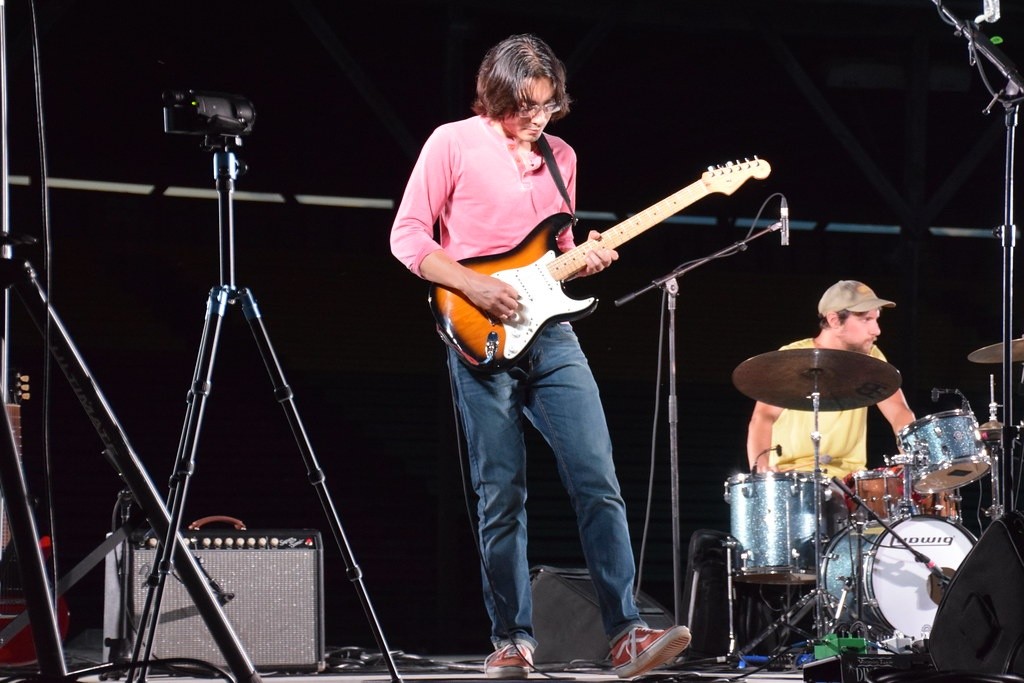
516,101 -> 559,117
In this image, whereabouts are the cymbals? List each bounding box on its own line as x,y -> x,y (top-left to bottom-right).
733,347 -> 902,412
967,339 -> 1024,363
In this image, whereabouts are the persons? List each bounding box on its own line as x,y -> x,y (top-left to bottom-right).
745,280 -> 936,569
388,33 -> 692,682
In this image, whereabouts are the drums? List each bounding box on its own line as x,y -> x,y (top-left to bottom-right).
821,516 -> 980,641
895,409 -> 993,495
841,465 -> 964,529
723,472 -> 850,586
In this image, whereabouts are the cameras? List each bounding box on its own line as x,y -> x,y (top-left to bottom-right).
161,88 -> 256,150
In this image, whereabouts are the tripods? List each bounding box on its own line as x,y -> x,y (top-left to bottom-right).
0,226 -> 267,683
123,152 -> 400,683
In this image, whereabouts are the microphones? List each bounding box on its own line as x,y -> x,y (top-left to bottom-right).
752,443 -> 783,476
931,387 -> 969,404
780,196 -> 790,246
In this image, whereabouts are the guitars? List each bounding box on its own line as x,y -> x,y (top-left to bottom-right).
427,155 -> 772,373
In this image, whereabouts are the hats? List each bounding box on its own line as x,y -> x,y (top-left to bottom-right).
817,280 -> 895,319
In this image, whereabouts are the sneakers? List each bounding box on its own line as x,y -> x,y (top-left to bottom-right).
610,624 -> 692,677
484,644 -> 535,679
